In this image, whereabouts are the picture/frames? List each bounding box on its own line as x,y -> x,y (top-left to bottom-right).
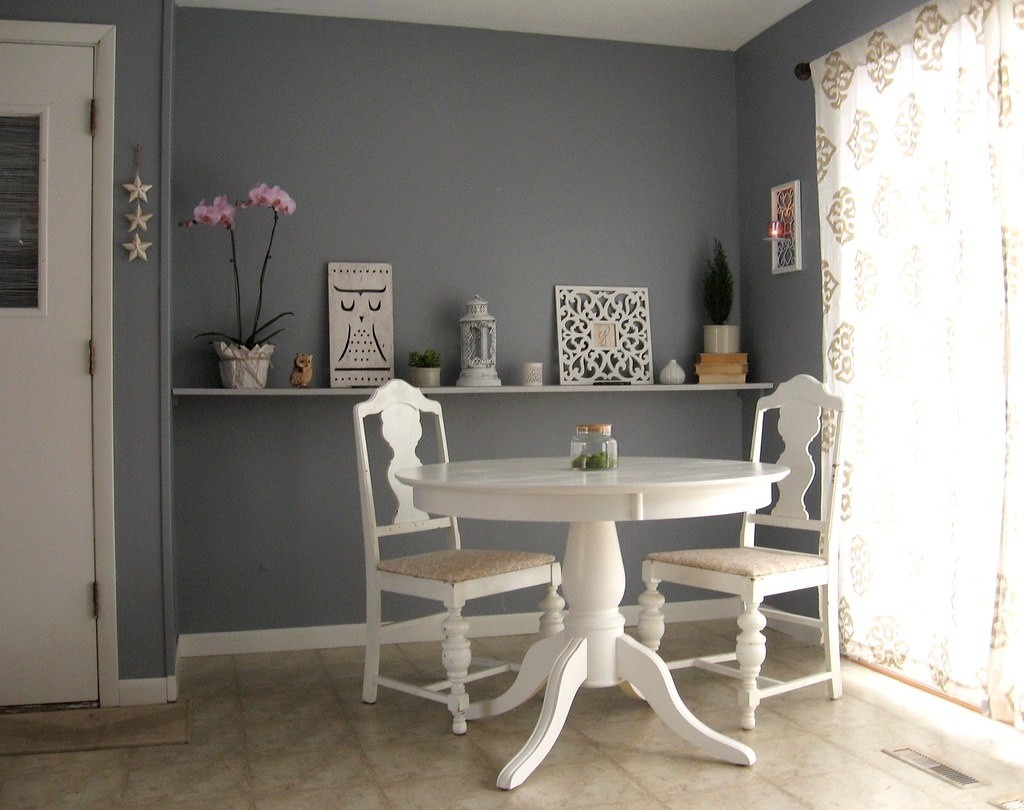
770,179 -> 802,275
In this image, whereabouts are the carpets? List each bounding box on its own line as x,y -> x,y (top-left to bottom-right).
0,700 -> 192,755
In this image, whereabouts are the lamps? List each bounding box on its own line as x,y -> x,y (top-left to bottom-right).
455,294 -> 502,387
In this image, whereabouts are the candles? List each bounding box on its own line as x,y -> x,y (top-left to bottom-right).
767,221 -> 784,238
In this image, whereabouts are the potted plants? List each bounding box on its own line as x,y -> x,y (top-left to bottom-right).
703,237 -> 740,353
407,347 -> 442,387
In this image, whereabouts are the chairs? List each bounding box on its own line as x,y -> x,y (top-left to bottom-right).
638,374 -> 846,731
352,379 -> 566,735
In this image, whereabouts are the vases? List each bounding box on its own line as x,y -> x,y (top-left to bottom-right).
660,359 -> 686,385
212,341 -> 276,389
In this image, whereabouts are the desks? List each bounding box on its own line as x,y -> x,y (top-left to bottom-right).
394,456 -> 792,791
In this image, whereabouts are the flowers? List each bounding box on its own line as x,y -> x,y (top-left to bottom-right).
177,183 -> 298,350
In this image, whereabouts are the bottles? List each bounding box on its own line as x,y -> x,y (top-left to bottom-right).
570,424 -> 618,469
